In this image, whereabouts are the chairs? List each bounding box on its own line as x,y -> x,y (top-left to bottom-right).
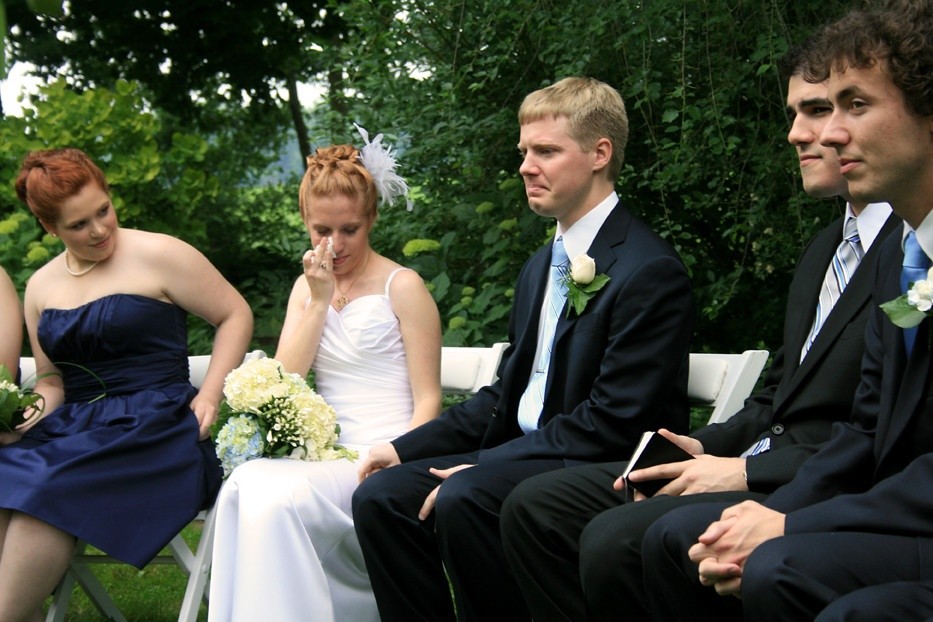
45,350 -> 269,622
439,341 -> 509,397
687,350 -> 771,426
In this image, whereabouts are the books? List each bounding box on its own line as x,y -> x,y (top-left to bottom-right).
622,431 -> 697,498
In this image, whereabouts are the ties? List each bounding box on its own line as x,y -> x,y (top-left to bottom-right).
739,217 -> 862,460
517,237 -> 571,433
900,230 -> 929,357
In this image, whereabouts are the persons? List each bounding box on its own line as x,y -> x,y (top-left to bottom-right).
579,55 -> 903,622
688,0 -> 933,622
0,149 -> 255,622
207,146 -> 442,622
351,77 -> 690,622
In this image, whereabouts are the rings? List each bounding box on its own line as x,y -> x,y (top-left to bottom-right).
319,264 -> 326,268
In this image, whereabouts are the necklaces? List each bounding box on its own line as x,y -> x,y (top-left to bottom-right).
336,252 -> 371,312
64,248 -> 100,277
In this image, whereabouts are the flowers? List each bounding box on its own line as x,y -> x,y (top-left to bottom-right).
558,253 -> 611,319
0,363 -> 108,433
208,354 -> 358,480
877,265 -> 933,328
